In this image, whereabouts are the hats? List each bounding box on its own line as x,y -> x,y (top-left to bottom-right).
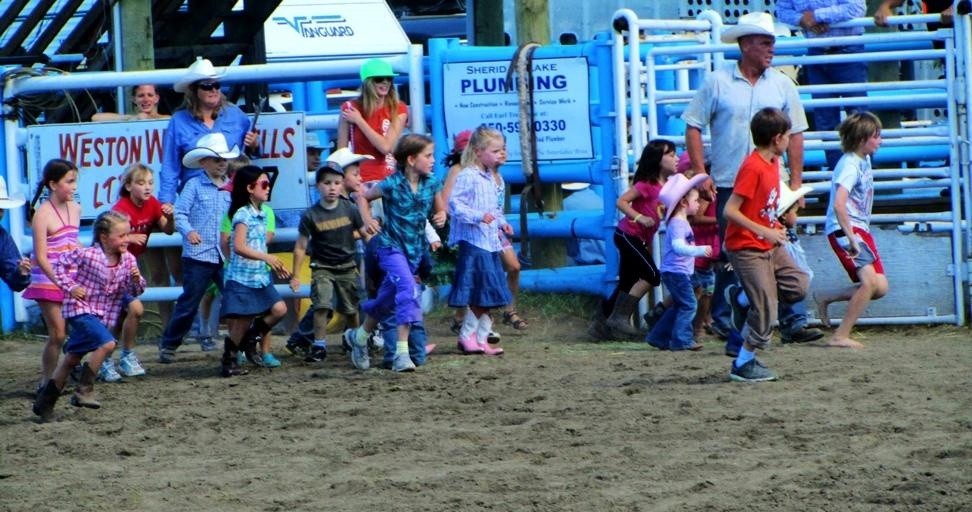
182,133 -> 240,169
0,177 -> 26,210
721,11 -> 791,44
360,58 -> 399,81
658,173 -> 709,222
173,59 -> 228,92
325,148 -> 375,173
778,180 -> 814,219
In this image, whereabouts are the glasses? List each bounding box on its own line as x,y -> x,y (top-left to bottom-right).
198,82 -> 222,90
252,180 -> 270,190
374,77 -> 392,83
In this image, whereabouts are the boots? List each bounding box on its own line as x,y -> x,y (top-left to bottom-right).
450,311 -> 527,354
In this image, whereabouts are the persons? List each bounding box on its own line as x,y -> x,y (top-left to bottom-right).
588,107 -> 811,384
812,112 -> 890,349
282,59 -> 523,372
776,1 -> 968,172
682,10 -> 827,344
1,52 -> 295,420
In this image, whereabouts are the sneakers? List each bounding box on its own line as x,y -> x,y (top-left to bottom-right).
726,356 -> 773,382
33,351 -> 146,415
159,334 -> 435,374
588,283 -> 821,355
